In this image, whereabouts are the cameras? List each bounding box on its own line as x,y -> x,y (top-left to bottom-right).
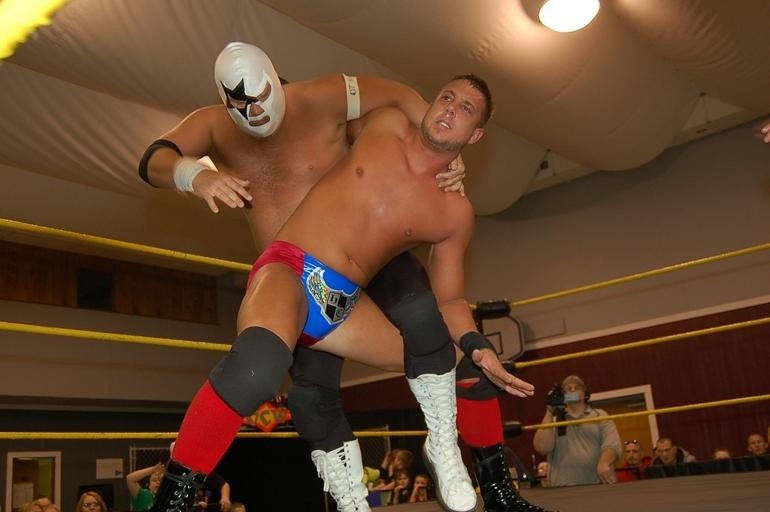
546,386 -> 579,407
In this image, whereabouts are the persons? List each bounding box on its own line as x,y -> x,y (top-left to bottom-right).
147,73 -> 557,512
357,449 -> 436,508
501,425 -> 770,489
21,441 -> 246,510
136,38 -> 481,512
532,373 -> 623,488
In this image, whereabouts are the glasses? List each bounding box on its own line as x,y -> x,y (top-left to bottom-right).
624,439 -> 641,445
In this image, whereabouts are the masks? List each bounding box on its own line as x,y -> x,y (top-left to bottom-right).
563,391 -> 589,404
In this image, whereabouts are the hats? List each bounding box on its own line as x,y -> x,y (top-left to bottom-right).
561,375 -> 587,389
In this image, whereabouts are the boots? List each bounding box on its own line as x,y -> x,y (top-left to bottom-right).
151,458 -> 210,511
310,437 -> 370,511
468,443 -> 560,511
405,365 -> 479,512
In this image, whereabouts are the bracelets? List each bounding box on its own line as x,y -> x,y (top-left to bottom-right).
458,330 -> 498,362
173,157 -> 210,195
